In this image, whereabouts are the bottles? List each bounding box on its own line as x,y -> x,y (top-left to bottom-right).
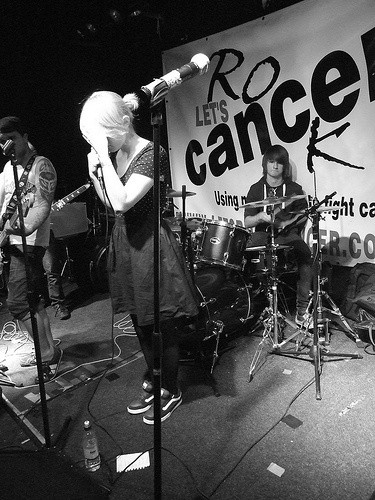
82,421 -> 101,472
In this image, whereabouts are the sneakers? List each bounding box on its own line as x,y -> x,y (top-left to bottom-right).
295,312 -> 324,329
35,349 -> 63,384
142,388 -> 182,424
53,303 -> 70,320
21,356 -> 37,366
127,381 -> 165,415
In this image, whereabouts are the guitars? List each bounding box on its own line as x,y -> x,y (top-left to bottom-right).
0,199 -> 31,276
51,179 -> 94,213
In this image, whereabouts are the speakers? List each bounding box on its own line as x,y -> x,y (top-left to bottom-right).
0,450 -> 112,500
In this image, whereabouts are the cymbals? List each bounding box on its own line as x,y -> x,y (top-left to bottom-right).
288,206 -> 346,213
164,216 -> 202,231
165,188 -> 196,198
239,194 -> 308,208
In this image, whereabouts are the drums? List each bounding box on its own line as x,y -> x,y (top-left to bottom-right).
91,244 -> 110,282
194,218 -> 252,272
189,265 -> 251,349
246,244 -> 298,278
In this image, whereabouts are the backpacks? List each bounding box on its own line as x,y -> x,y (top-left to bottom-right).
340,261 -> 375,321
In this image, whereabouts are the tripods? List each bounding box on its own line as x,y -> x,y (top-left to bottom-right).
246,191 -> 364,400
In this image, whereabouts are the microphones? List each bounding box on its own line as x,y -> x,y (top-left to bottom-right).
137,53 -> 210,102
97,164 -> 104,190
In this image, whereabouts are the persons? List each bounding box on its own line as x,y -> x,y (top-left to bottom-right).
0,116 -> 71,385
79,90 -> 200,425
244,145 -> 315,330
